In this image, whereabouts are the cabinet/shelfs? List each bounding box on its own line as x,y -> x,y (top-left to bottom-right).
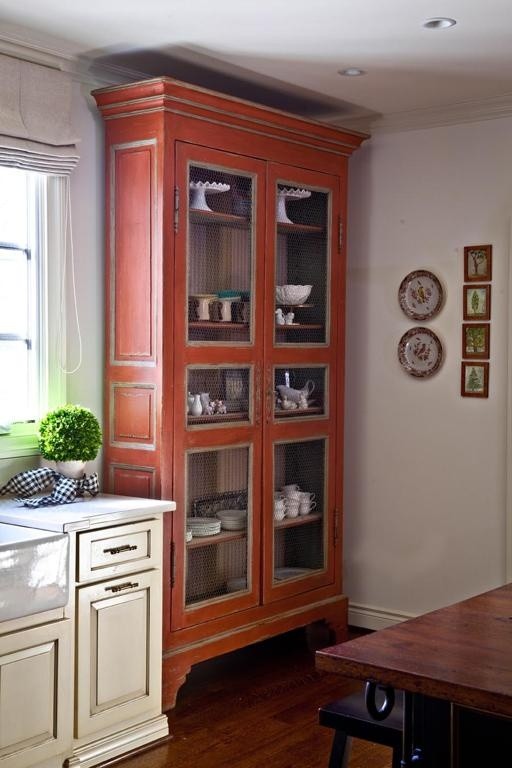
89,74 -> 373,712
65,511 -> 170,767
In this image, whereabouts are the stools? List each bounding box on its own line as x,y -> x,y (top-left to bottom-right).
317,686 -> 403,768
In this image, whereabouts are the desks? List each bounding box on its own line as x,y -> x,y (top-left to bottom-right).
315,581 -> 512,768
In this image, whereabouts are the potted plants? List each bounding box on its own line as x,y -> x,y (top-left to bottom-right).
38,402 -> 103,479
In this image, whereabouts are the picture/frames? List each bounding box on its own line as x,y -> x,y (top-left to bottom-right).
461,244 -> 492,398
